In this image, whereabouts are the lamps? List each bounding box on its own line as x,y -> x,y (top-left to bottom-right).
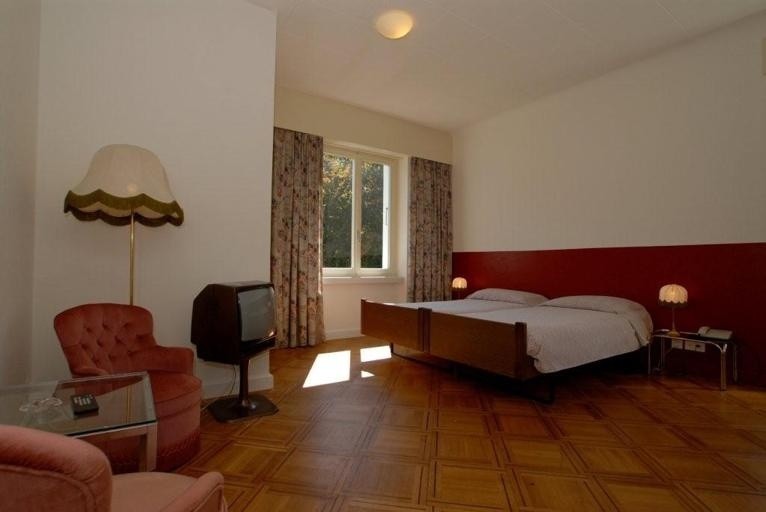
451,276 -> 468,299
64,141 -> 185,306
371,5 -> 417,43
656,283 -> 690,337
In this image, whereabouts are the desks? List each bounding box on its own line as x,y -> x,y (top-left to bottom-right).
650,331 -> 740,392
193,338 -> 280,424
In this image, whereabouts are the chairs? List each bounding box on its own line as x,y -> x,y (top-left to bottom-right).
58,302 -> 203,474
0,422 -> 228,512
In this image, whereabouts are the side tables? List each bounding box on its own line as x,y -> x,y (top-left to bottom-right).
0,369 -> 160,473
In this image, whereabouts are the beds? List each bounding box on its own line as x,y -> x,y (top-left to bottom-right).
359,288 -> 650,407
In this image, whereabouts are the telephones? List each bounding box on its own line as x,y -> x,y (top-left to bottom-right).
698,326 -> 733,340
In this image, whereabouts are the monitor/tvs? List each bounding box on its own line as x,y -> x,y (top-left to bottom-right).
190,281 -> 277,366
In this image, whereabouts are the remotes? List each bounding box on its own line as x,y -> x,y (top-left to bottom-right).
70,392 -> 99,414
